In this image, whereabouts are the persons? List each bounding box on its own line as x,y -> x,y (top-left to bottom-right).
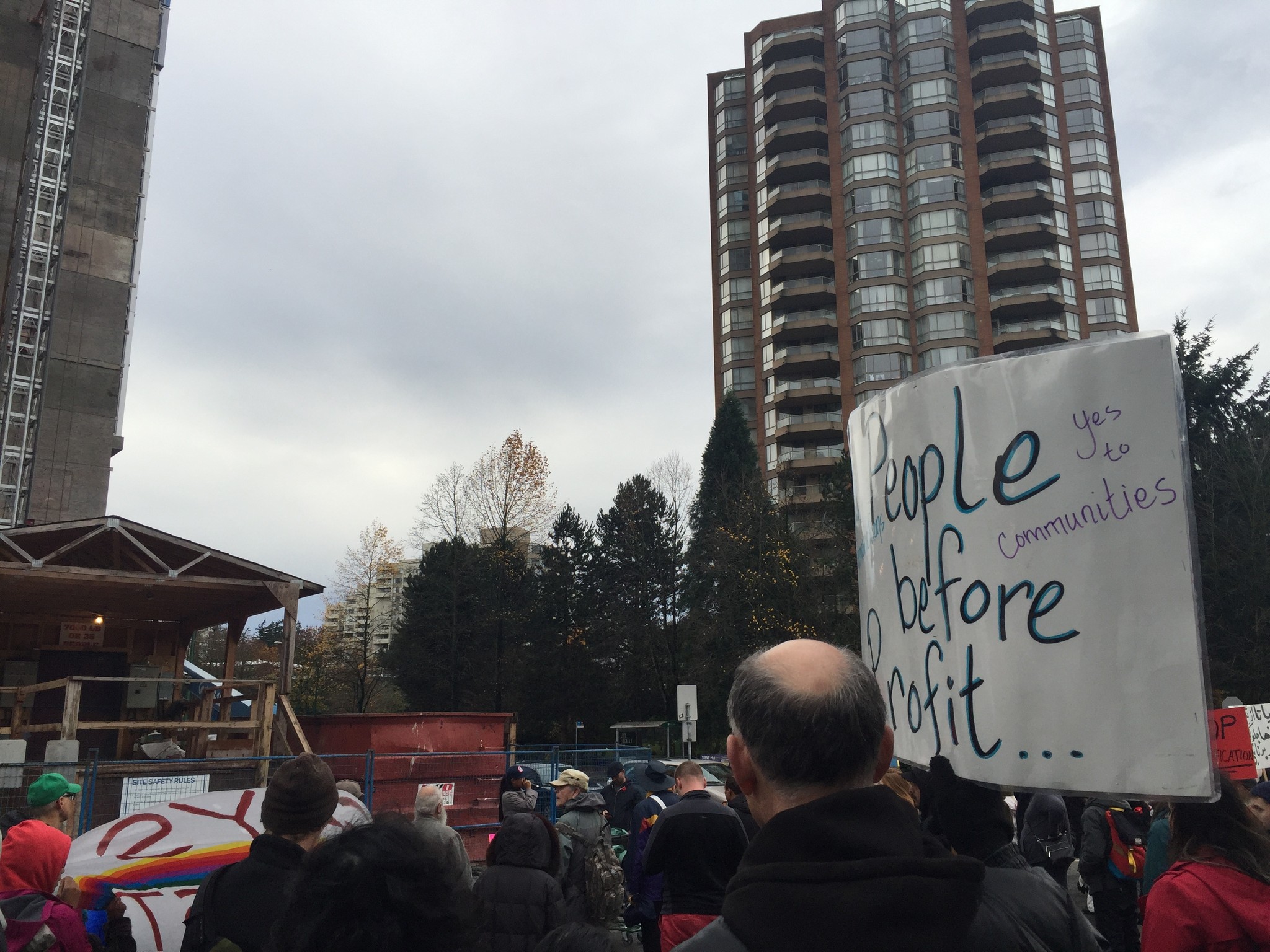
466,813 -> 572,952
641,761 -> 752,951
599,762 -> 646,836
180,755 -> 346,952
551,767 -> 615,937
501,765 -> 539,821
0,820 -> 99,952
622,765 -> 680,952
723,776 -> 762,844
409,785 -> 474,895
27,770 -> 84,840
672,639 -> 1106,952
875,737 -> 1270,952
76,888 -> 138,952
250,811 -> 468,952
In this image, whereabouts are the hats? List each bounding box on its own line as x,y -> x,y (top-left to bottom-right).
336,779 -> 365,799
260,752 -> 339,835
505,766 -> 525,780
1239,777 -> 1258,789
607,761 -> 623,778
27,772 -> 82,807
549,768 -> 589,793
634,759 -> 676,791
1251,780 -> 1270,802
900,766 -> 929,784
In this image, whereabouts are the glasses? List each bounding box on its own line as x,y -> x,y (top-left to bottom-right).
56,793 -> 75,804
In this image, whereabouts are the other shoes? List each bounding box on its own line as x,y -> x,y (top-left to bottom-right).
1082,906 -> 1096,918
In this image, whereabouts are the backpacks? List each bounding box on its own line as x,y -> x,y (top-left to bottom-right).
1089,801 -> 1146,886
552,820 -> 625,927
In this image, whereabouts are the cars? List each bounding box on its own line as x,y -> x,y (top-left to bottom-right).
606,758 -> 733,806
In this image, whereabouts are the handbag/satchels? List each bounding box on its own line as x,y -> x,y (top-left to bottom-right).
1036,832 -> 1075,866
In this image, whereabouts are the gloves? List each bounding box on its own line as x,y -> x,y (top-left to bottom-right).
930,756 -> 1013,859
